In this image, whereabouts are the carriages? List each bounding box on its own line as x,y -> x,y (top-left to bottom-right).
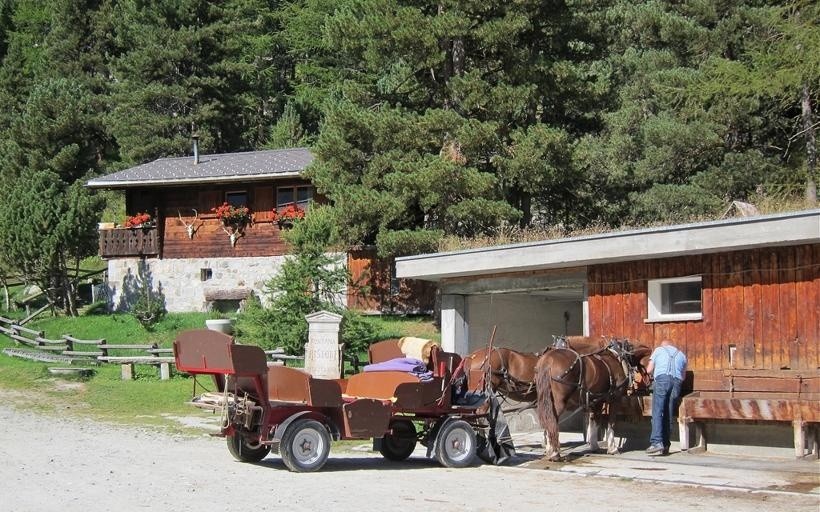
173,328 -> 652,471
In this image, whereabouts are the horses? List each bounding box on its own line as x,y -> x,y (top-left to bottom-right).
462,347 -> 547,448
551,334 -> 641,354
534,344 -> 653,462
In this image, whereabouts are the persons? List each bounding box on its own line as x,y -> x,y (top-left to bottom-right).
645,338 -> 688,455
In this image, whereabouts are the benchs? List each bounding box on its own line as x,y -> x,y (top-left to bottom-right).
175,330 -> 464,407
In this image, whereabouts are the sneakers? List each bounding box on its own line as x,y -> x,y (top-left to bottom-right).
646,442 -> 669,454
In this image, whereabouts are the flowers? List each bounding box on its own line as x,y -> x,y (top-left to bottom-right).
124,212 -> 153,229
211,202 -> 251,227
270,202 -> 307,223
